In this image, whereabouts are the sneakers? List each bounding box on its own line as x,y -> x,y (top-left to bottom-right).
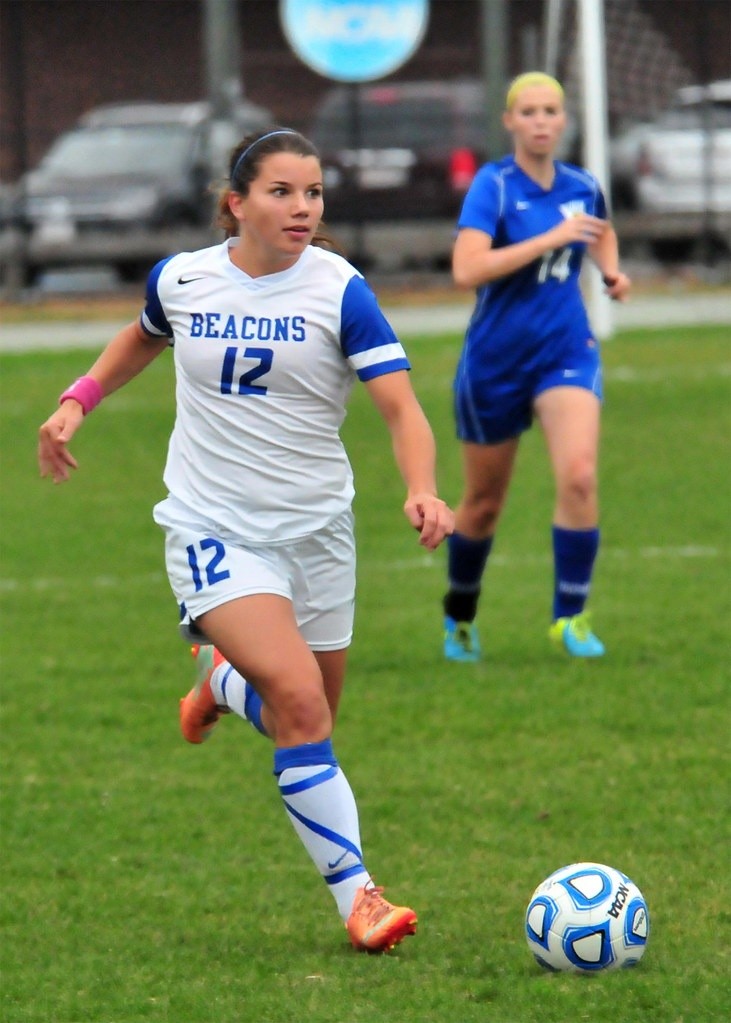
444,613 -> 480,663
348,885 -> 418,954
549,609 -> 605,658
181,642 -> 233,744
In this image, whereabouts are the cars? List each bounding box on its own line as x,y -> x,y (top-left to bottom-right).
637,77 -> 731,276
13,98 -> 272,283
303,75 -> 487,272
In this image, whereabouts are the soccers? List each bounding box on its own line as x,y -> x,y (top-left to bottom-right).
524,863 -> 649,975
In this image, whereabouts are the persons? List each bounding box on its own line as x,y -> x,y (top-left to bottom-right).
37,122 -> 464,951
441,70 -> 631,663
